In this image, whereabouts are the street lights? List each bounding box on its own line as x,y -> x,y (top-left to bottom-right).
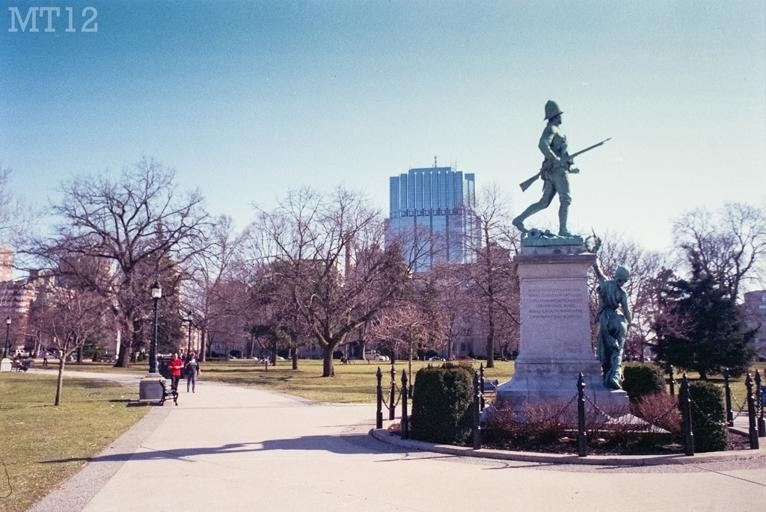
187,310 -> 193,357
2,315 -> 12,357
148,279 -> 161,372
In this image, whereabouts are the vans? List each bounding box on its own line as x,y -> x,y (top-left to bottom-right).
362,349 -> 390,360
332,351 -> 352,360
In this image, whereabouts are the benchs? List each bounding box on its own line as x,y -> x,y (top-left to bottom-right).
158,380 -> 179,406
9,361 -> 25,373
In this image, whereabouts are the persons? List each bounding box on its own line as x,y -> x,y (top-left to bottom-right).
41,350 -> 49,367
13,353 -> 27,372
340,356 -> 350,363
509,97 -> 578,239
184,350 -> 200,394
168,352 -> 183,395
586,227 -> 632,389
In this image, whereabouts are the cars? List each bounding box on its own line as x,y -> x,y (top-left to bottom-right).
429,356 -> 444,360
14,349 -> 56,359
616,355 -> 658,362
750,351 -> 766,361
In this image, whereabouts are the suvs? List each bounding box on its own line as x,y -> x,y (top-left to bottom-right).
101,354 -> 116,361
